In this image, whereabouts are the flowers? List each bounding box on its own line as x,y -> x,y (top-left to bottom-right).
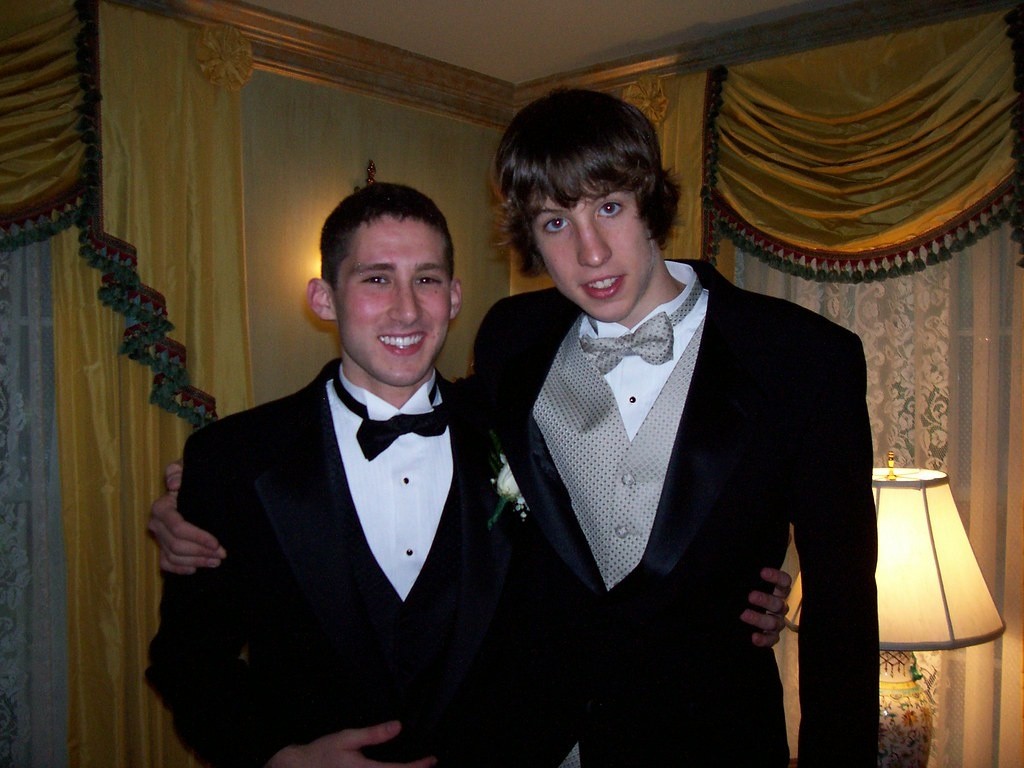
485,429 -> 530,530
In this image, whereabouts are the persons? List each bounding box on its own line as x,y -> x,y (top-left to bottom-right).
144,183 -> 793,768
143,89 -> 884,767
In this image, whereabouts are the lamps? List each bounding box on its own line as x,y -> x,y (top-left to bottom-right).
784,451 -> 1008,768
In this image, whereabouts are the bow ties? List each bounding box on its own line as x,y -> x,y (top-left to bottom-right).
334,361 -> 451,462
578,274 -> 703,376
